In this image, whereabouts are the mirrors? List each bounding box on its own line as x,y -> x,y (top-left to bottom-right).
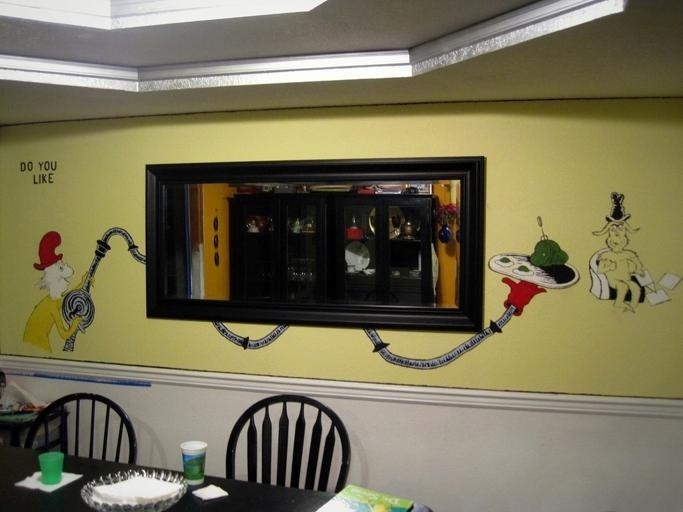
139,156 -> 487,332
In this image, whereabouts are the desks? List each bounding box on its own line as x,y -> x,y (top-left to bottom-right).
0,444 -> 427,510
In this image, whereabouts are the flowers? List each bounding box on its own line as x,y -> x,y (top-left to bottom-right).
435,204 -> 457,226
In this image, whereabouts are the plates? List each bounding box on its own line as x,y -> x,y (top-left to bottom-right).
81,468 -> 188,511
368,206 -> 406,239
345,241 -> 370,271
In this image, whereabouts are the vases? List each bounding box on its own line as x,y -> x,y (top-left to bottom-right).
438,222 -> 454,243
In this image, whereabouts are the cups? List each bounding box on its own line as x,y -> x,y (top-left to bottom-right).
179,440 -> 209,486
38,450 -> 65,485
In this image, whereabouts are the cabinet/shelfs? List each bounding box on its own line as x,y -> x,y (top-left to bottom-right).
228,188 -> 437,308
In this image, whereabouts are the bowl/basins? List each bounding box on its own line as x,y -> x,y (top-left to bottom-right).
362,268 -> 376,278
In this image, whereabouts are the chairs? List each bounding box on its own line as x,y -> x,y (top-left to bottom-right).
19,388 -> 140,467
222,391 -> 352,489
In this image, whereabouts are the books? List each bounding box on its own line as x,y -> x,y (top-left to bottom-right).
315,483 -> 414,512
